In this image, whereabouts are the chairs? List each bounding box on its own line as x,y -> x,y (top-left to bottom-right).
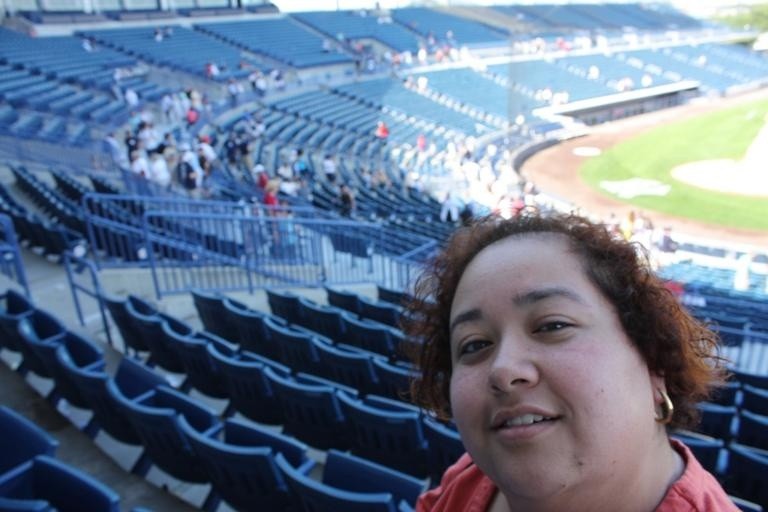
0,0 -> 768,511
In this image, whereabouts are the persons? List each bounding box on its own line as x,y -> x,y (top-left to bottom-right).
397,208 -> 745,511
74,3 -> 707,252
